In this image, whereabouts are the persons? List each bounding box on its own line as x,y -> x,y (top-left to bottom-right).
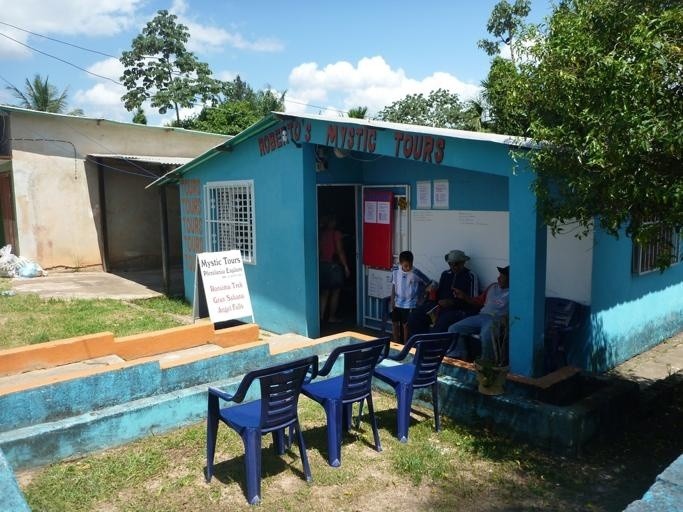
447,266 -> 509,362
390,251 -> 430,343
318,214 -> 351,324
407,250 -> 481,345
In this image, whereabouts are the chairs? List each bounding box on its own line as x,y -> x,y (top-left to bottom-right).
207,355 -> 319,505
288,337 -> 390,467
355,332 -> 458,444
545,297 -> 583,366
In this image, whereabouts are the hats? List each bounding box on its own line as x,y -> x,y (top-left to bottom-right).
444,250 -> 470,263
497,266 -> 509,276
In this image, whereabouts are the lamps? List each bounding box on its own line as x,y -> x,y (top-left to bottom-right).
282,126 -> 302,148
